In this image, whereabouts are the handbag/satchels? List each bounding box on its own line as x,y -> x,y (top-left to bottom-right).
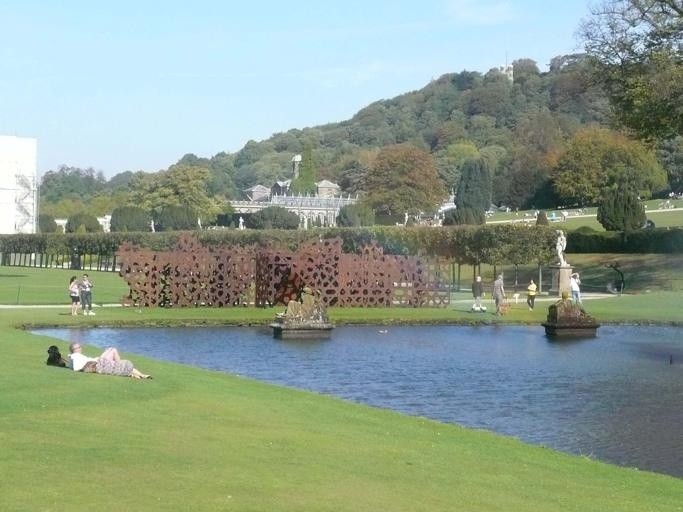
70,289 -> 78,296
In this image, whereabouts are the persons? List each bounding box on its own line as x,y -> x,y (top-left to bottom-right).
569,272 -> 582,303
275,287 -> 322,317
472,276 -> 485,308
68,274 -> 96,317
527,279 -> 538,312
68,343 -> 151,379
492,274 -> 506,314
555,229 -> 567,266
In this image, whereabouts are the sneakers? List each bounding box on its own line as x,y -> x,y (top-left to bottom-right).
83,310 -> 95,316
141,375 -> 153,380
